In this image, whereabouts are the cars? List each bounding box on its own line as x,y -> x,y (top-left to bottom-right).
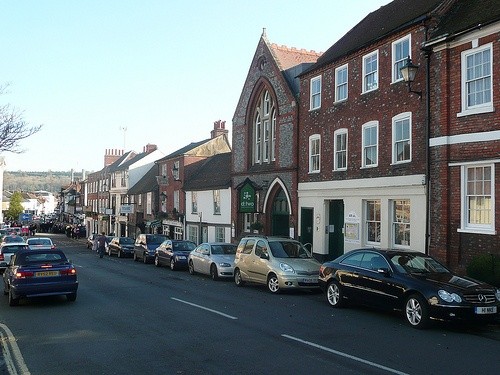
86,234 -> 104,249
154,239 -> 199,272
95,236 -> 118,255
26,237 -> 56,250
0,222 -> 30,244
108,237 -> 137,258
0,246 -> 79,307
317,246 -> 500,329
187,242 -> 239,281
0,242 -> 29,275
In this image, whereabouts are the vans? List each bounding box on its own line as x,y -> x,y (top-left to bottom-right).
232,234 -> 323,294
133,233 -> 174,265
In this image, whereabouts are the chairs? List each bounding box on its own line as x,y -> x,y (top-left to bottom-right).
398,257 -> 412,271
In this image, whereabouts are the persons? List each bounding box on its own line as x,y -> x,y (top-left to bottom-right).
98,232 -> 108,259
11,220 -> 85,239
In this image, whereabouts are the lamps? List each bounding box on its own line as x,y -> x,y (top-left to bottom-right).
173,164 -> 181,181
400,55 -> 422,99
